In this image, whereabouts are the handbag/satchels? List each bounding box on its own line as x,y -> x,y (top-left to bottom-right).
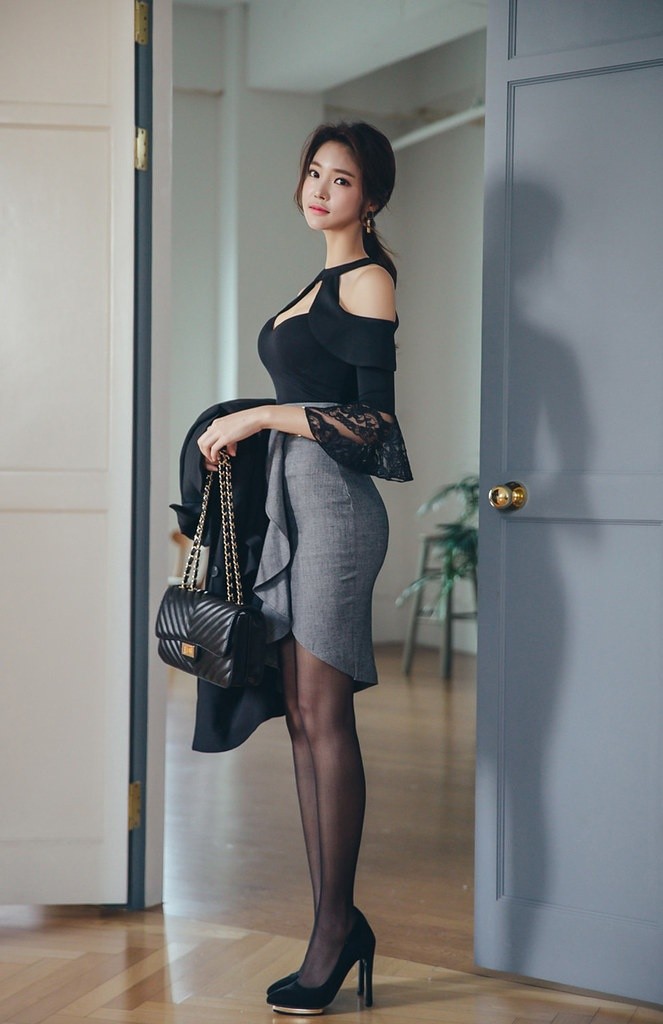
155,449 -> 267,691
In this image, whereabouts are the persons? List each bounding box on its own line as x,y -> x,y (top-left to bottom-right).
196,121 -> 415,1017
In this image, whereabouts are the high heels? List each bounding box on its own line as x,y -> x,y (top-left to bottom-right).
265,905 -> 376,1015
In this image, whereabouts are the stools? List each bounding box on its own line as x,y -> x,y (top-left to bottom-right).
398,532 -> 478,681
